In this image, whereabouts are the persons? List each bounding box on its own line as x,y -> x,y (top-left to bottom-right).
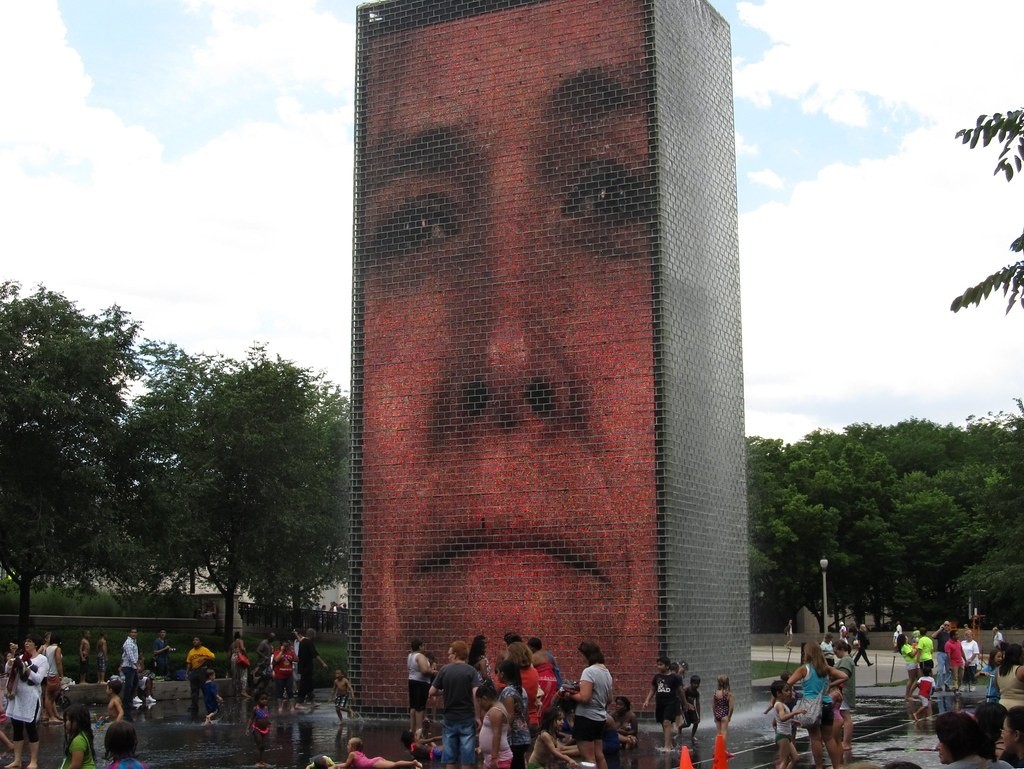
763,619 -> 1024,769
332,736 -> 424,769
713,674 -> 736,757
1,594 -> 355,769
400,631 -> 704,768
357,0 -> 650,706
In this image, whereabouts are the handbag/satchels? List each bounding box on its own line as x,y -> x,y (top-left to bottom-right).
792,699 -> 821,728
152,657 -> 157,667
46,674 -> 62,692
546,651 -> 562,689
237,651 -> 250,669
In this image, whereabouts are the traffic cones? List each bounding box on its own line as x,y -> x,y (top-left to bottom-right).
679,746 -> 695,769
712,735 -> 729,769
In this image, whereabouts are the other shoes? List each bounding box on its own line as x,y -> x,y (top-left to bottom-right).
133,696 -> 143,702
726,752 -> 736,758
145,695 -> 156,702
868,663 -> 874,666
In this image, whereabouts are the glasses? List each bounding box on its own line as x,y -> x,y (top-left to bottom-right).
999,729 -> 1013,736
945,625 -> 949,626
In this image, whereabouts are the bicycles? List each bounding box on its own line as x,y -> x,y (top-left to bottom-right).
54,685 -> 70,714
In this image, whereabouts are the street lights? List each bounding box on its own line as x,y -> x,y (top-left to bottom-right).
820,554 -> 829,635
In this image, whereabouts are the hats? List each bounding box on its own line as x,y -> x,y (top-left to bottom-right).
679,660 -> 688,670
839,622 -> 844,626
